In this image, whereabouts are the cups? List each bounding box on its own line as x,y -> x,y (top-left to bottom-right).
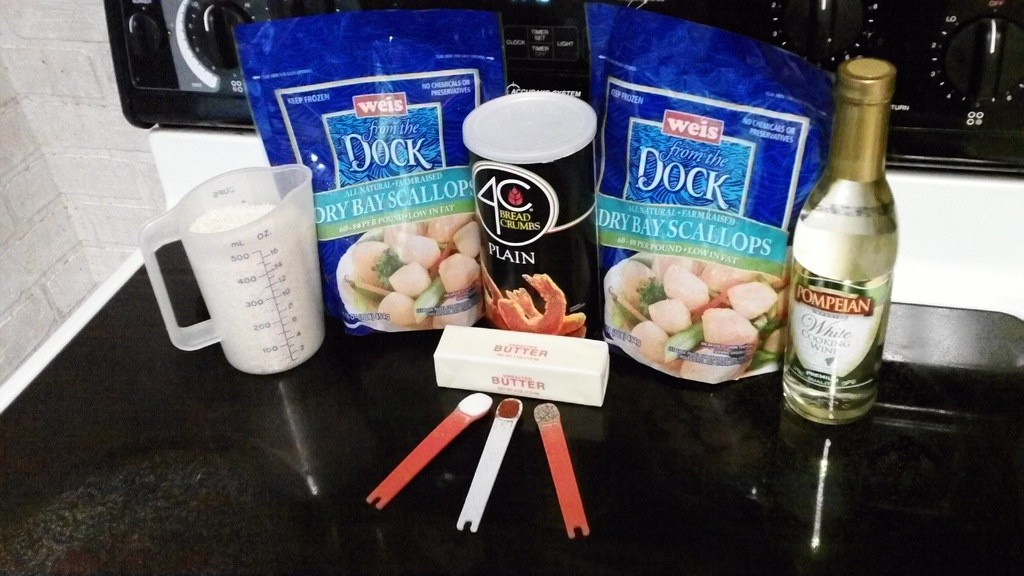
139,163 -> 325,374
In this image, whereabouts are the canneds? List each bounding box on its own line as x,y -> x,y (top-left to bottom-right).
463,91 -> 602,341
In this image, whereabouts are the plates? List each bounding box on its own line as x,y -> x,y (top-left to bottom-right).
604,247 -> 802,382
337,214 -> 490,332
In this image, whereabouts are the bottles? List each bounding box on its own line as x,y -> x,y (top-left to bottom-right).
783,58 -> 899,424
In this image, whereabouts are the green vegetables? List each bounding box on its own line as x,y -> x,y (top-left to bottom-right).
346,239 -> 453,323
612,278 -> 786,374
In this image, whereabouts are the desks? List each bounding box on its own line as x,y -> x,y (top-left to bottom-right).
0,235 -> 1024,576
